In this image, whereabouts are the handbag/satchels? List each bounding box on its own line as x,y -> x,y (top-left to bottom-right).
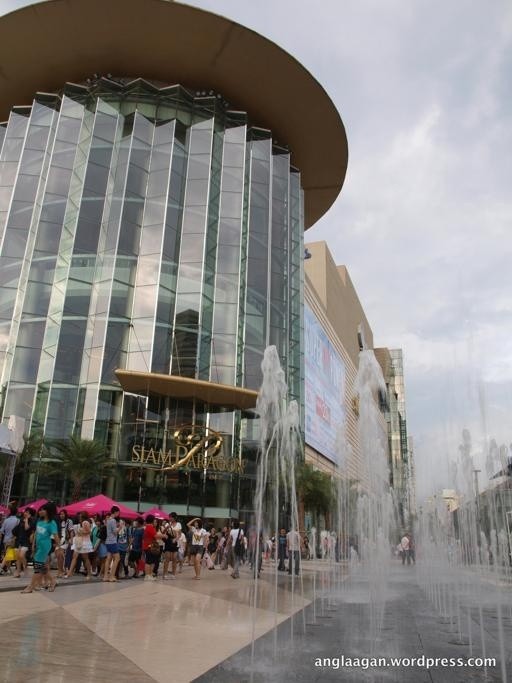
205,552 -> 215,570
65,528 -> 74,542
150,542 -> 161,555
96,526 -> 107,540
234,540 -> 246,557
5,548 -> 16,561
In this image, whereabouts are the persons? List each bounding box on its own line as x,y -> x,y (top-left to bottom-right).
390,533 -> 416,564
1,502 -> 312,593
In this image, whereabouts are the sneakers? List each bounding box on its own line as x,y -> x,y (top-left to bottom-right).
143,574 -> 175,581
83,575 -> 130,582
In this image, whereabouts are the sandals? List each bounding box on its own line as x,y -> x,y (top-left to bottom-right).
21,581 -> 58,592
